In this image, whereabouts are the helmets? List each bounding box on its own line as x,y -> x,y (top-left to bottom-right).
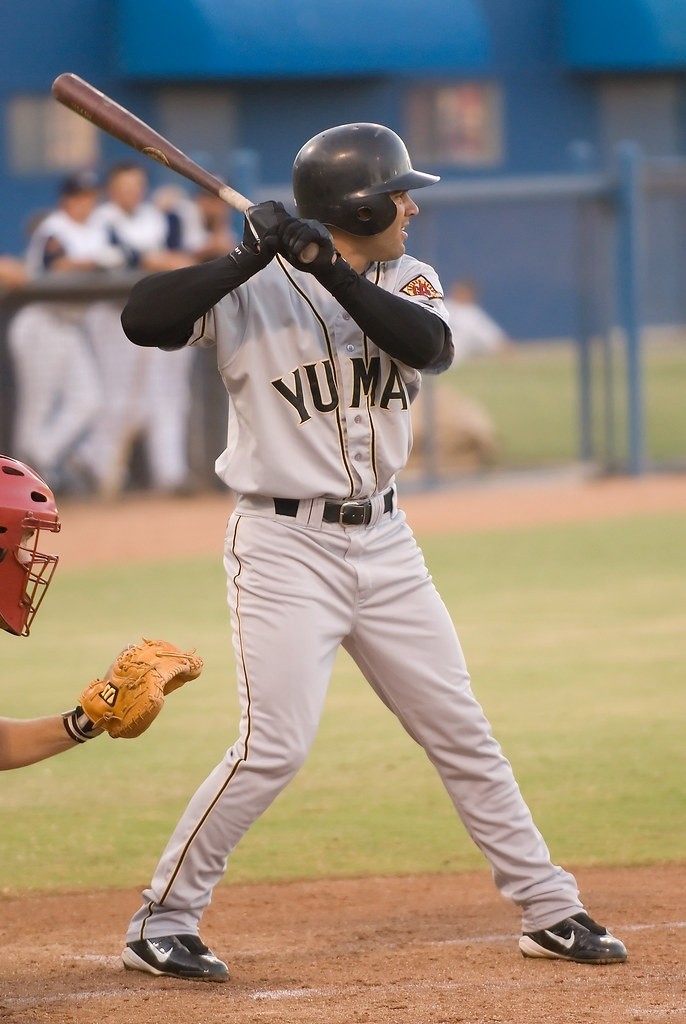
0,452 -> 62,634
293,123 -> 441,237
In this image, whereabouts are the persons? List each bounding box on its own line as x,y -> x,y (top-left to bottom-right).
0,454 -> 202,771
119,122 -> 629,983
11,166 -> 507,498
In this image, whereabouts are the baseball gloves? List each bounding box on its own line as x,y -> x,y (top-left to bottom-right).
77,638 -> 205,738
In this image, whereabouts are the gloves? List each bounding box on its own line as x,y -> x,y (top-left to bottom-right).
277,217 -> 342,276
228,201 -> 295,277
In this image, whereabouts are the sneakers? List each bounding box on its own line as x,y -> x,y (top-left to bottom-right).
121,934 -> 230,982
519,913 -> 627,963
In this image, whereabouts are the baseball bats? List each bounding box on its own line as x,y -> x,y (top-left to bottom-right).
52,73 -> 320,264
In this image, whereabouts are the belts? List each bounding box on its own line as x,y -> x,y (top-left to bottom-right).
274,491 -> 394,527
